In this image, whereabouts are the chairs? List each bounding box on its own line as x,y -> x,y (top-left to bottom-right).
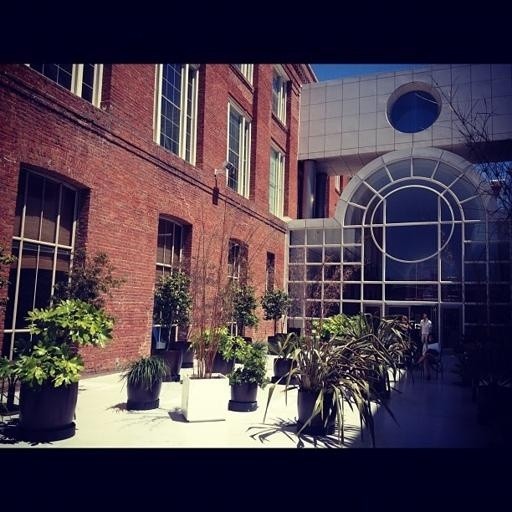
424,342 -> 447,381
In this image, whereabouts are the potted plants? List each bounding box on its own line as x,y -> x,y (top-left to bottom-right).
175,215 -> 268,423
121,357 -> 166,411
150,270 -> 193,382
2,293 -> 115,441
263,286 -> 398,436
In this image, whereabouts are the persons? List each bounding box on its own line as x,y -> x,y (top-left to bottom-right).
413,347 -> 441,381
419,313 -> 432,350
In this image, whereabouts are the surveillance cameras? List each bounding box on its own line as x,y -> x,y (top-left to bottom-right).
214,160 -> 238,177
